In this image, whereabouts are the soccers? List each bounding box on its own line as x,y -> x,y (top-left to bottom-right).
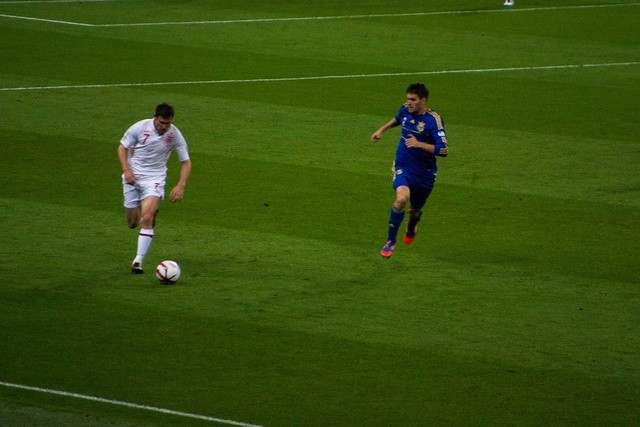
155,260 -> 181,283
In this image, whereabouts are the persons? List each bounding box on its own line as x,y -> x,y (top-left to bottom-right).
118,103 -> 192,274
371,83 -> 449,259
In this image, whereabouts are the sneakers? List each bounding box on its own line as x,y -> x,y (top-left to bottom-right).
131,260 -> 144,273
404,223 -> 417,244
152,209 -> 159,228
380,239 -> 395,257
